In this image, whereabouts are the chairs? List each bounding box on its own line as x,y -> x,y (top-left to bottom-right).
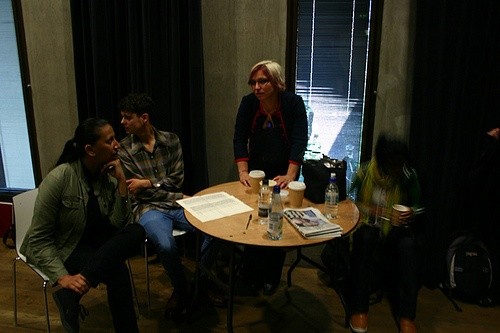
11,163 -> 200,333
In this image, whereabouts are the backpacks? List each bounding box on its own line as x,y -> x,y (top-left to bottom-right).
447,234 -> 499,307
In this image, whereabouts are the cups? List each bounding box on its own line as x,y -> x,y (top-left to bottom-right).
250,170 -> 264,192
260,180 -> 276,192
390,204 -> 409,225
287,181 -> 306,209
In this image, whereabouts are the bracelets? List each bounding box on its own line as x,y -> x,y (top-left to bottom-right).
238,170 -> 249,172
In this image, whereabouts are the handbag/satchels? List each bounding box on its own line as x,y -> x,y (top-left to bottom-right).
302,153 -> 347,204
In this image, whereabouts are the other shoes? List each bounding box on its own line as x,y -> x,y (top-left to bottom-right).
400,318 -> 416,333
199,289 -> 232,307
263,271 -> 280,294
350,313 -> 368,332
165,284 -> 192,318
52,287 -> 90,333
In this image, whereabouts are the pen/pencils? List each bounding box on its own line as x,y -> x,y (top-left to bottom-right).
246,214 -> 252,230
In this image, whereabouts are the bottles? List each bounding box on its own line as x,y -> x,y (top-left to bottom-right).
256,178 -> 271,224
267,185 -> 283,239
322,173 -> 338,219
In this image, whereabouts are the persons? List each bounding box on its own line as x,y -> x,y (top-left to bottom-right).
342,136 -> 425,333
19,116 -> 139,333
234,60 -> 308,296
117,92 -> 221,327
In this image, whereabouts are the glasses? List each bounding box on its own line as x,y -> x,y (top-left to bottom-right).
248,78 -> 271,85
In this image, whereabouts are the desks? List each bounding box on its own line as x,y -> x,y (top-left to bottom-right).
183,178 -> 363,333
